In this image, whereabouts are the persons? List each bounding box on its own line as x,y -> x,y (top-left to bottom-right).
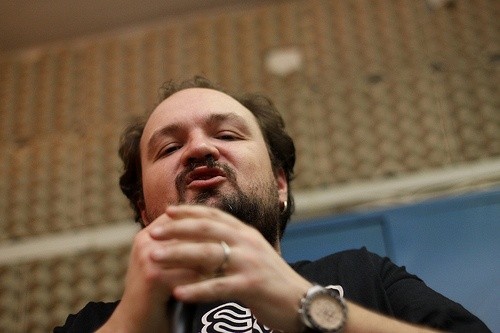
46,71 -> 494,333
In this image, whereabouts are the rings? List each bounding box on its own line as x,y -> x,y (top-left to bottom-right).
211,240 -> 232,279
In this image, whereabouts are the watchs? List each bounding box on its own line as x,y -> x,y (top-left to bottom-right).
295,283 -> 350,333
